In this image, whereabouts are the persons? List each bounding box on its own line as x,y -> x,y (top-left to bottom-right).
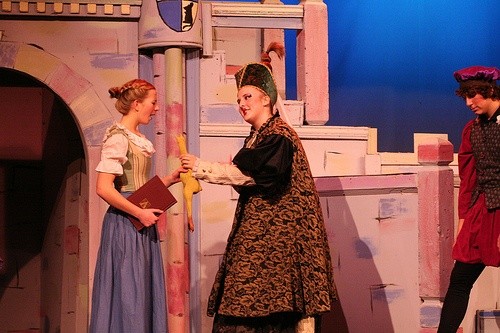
180,60 -> 339,332
439,65 -> 500,332
88,79 -> 190,333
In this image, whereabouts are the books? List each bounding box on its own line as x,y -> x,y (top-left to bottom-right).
124,175 -> 178,231
